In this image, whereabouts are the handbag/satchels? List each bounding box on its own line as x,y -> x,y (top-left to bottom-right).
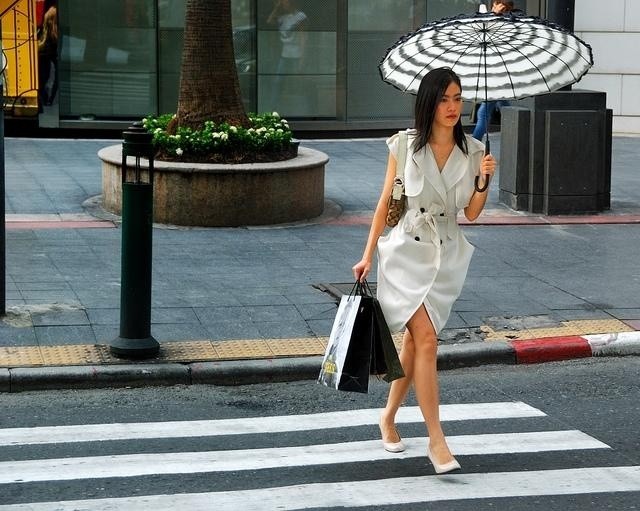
318,281 -> 406,392
385,177 -> 405,228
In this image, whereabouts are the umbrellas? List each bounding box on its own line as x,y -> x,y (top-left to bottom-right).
378,12 -> 593,191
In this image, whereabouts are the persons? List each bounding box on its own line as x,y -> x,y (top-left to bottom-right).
37,6 -> 63,107
353,66 -> 496,475
318,299 -> 352,389
472,1 -> 511,141
266,0 -> 318,115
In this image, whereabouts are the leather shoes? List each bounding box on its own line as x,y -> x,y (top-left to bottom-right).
426,443 -> 461,474
377,416 -> 405,452
